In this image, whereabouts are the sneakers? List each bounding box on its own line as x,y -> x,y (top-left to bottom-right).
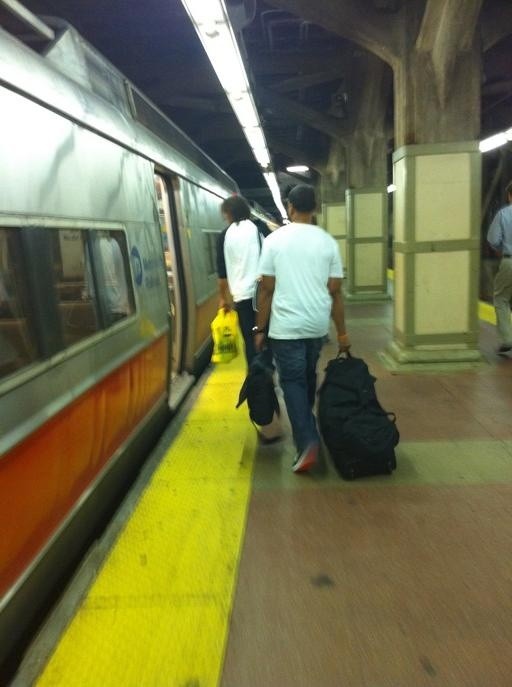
291,440 -> 320,472
495,345 -> 511,354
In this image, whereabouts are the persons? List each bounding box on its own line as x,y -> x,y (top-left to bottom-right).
216,194 -> 273,369
484,181 -> 512,354
0,228 -> 131,376
250,181 -> 355,473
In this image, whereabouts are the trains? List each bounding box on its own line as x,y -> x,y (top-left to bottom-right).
2,1 -> 286,675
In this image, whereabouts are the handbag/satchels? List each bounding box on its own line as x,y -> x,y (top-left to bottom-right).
210,308 -> 242,365
236,353 -> 288,446
318,350 -> 398,476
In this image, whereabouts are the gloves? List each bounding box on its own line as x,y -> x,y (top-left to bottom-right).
337,333 -> 351,352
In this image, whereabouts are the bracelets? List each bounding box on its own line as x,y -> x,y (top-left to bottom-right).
247,325 -> 266,337
337,333 -> 348,340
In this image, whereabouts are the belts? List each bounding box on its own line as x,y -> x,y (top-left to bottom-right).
502,254 -> 512,258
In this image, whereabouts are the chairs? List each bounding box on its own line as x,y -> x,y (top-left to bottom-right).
1,282 -> 101,380
